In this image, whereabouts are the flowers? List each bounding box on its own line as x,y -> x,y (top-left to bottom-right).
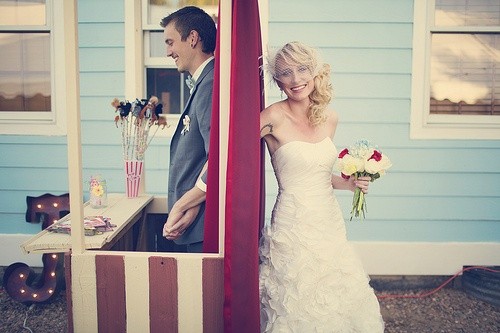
336,140 -> 392,222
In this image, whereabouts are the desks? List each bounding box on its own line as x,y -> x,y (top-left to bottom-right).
21,193 -> 155,255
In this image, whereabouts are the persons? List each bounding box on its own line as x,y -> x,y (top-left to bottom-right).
162,39 -> 372,333
160,6 -> 218,252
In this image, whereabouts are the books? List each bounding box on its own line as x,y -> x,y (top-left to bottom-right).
48,215 -> 118,236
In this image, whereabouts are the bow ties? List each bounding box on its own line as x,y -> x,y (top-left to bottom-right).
185,76 -> 195,90
